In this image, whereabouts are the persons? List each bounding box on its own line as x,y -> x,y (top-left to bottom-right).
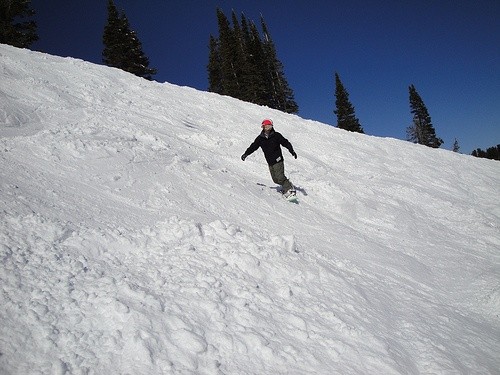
241,118 -> 298,196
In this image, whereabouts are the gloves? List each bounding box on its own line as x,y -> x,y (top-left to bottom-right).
291,151 -> 297,159
241,153 -> 247,161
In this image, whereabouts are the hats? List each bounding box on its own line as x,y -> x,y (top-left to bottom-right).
261,119 -> 273,128
